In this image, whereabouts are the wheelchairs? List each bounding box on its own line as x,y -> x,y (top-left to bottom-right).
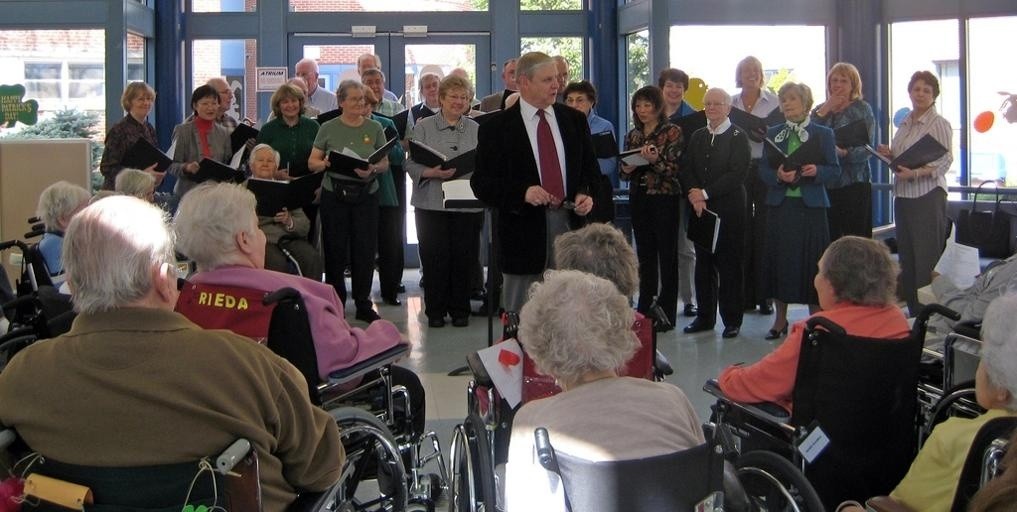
520,425 -> 825,512
0,241 -> 72,365
455,299 -> 673,512
706,302 -> 943,511
919,281 -> 1017,511
1,404 -> 413,512
174,271 -> 426,495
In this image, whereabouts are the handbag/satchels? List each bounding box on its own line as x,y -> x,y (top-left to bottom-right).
330,178 -> 370,204
956,180 -> 1016,258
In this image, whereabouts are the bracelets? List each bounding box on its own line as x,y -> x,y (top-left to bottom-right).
835,500 -> 863,512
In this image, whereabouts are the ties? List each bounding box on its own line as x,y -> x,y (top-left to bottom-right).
535,109 -> 565,210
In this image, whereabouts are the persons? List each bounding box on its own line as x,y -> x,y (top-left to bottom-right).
620,57 -> 876,339
712,236 -> 911,431
834,294 -> 1016,512
876,71 -> 953,319
101,53 -> 521,330
550,56 -> 617,223
171,175 -> 443,512
1,194 -> 348,512
494,266 -> 711,512
38,180 -> 92,276
474,223 -> 653,427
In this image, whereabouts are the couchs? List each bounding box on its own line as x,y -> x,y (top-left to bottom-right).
873,200 -> 1017,276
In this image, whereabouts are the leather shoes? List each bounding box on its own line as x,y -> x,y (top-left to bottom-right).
683,308 -> 791,339
355,284 -> 471,327
